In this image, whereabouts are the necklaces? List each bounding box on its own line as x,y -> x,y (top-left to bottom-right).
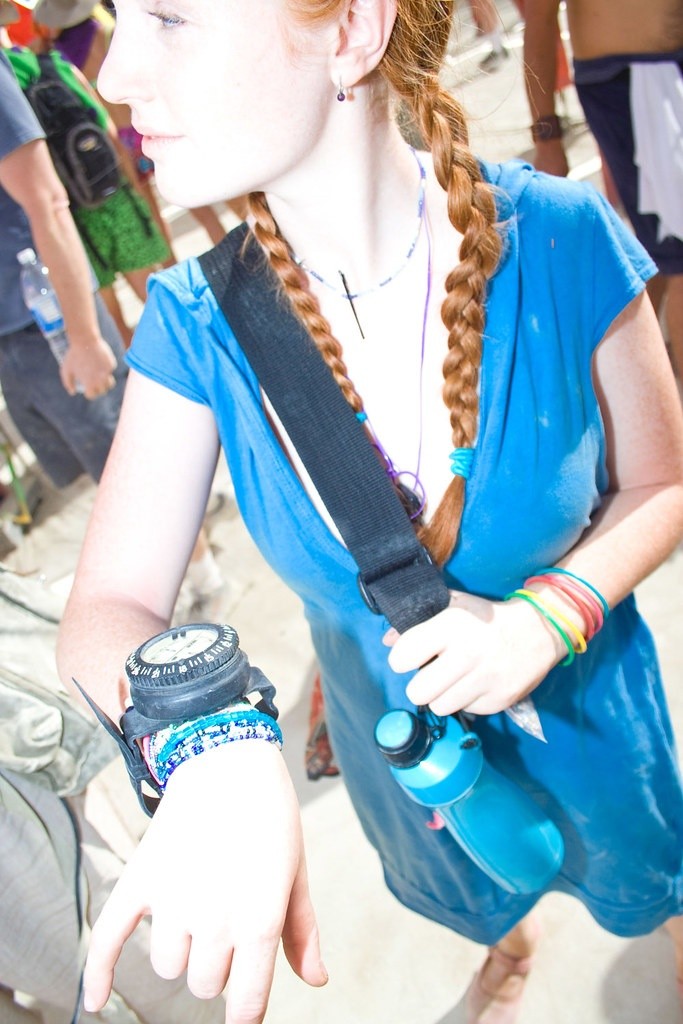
284,143 -> 427,293
316,237 -> 441,510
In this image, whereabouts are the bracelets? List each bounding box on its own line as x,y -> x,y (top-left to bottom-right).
144,703 -> 293,796
529,113 -> 564,142
499,565 -> 605,666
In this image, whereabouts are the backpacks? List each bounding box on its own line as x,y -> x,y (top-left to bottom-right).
17,48 -> 127,209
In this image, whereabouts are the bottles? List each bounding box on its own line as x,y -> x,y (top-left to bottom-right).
16,248 -> 86,393
374,710 -> 565,896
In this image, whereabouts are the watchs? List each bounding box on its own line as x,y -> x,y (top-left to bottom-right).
73,621 -> 282,818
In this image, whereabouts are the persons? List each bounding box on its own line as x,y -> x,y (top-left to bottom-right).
0,0 -> 682,620
58,0 -> 683,1024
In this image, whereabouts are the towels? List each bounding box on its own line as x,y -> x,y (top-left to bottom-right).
627,58 -> 683,245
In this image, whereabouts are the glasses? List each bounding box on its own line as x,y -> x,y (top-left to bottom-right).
371,442 -> 426,522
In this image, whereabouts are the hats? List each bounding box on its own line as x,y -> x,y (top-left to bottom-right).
33,0 -> 102,28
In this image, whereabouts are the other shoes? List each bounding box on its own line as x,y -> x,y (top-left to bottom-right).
201,491 -> 239,539
462,944 -> 536,1023
182,546 -> 224,605
